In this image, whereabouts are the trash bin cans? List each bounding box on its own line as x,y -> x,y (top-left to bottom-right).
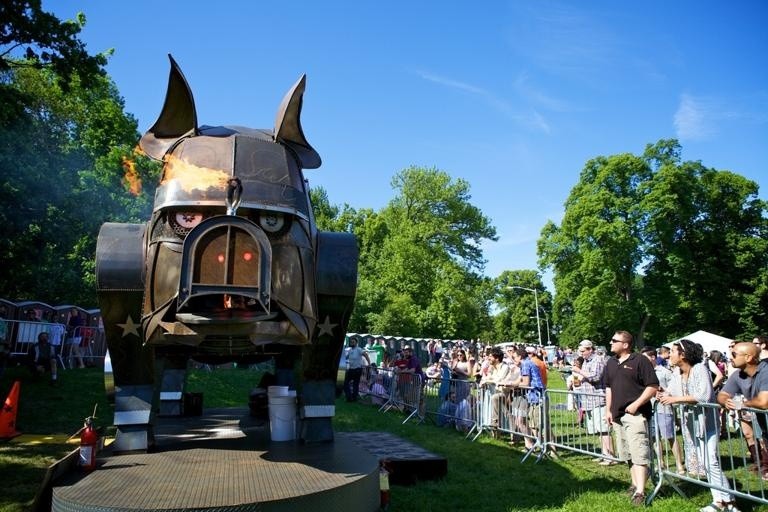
183,392 -> 203,417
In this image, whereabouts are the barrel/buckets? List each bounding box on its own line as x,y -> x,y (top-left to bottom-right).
268,395 -> 298,442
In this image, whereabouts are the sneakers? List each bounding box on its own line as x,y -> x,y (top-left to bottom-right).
591,458 -> 618,466
700,501 -> 741,512
507,441 -> 557,459
625,485 -> 646,505
678,466 -> 706,479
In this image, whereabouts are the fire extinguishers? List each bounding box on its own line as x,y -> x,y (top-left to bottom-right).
80,417 -> 97,471
379,460 -> 390,509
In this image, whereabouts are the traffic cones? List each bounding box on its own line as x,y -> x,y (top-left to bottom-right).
1,380 -> 23,440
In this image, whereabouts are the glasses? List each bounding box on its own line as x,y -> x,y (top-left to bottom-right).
728,345 -> 734,348
675,341 -> 685,352
732,352 -> 747,358
612,339 -> 623,343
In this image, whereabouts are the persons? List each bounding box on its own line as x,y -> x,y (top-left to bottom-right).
42,308 -> 55,323
79,320 -> 96,365
63,308 -> 85,369
0,305 -> 12,373
22,309 -> 41,352
25,331 -> 58,387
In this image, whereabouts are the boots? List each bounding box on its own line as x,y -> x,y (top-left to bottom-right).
747,444 -> 768,480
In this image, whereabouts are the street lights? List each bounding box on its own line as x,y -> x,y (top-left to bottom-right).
505,284 -> 544,348
528,313 -> 553,349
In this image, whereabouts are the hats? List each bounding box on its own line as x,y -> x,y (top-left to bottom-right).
525,346 -> 538,354
579,340 -> 592,347
